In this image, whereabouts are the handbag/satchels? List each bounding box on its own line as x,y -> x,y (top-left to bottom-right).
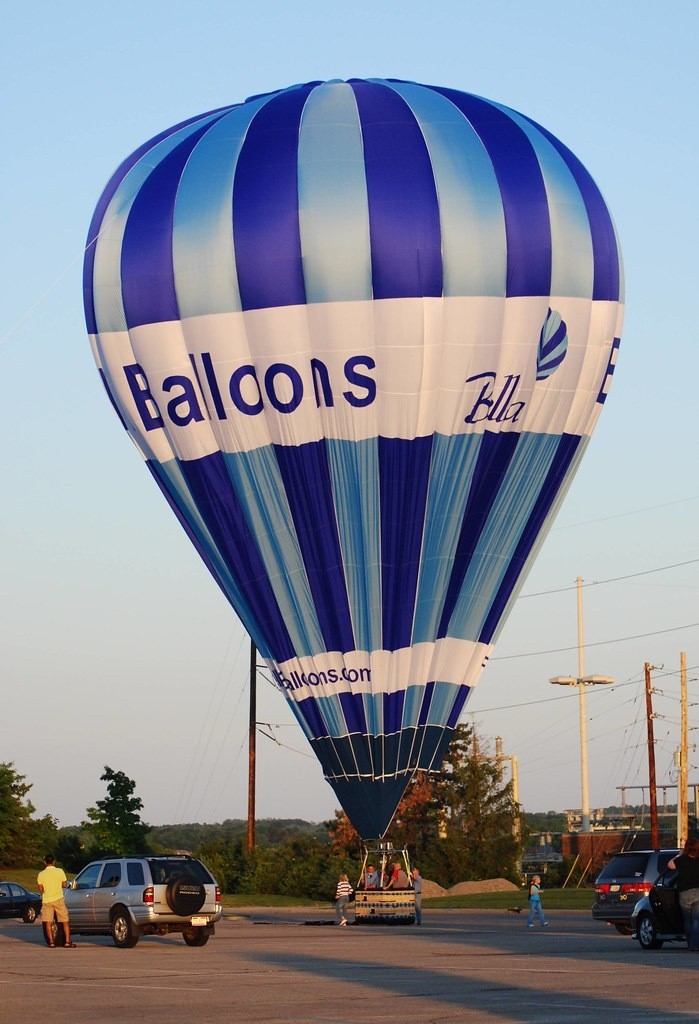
528,884 -> 540,900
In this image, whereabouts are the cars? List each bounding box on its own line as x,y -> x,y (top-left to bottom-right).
629,866 -> 687,950
0,882 -> 43,924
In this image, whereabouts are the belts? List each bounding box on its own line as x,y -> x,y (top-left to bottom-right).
414,891 -> 422,895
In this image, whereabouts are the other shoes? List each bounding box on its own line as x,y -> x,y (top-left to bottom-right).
417,921 -> 421,924
542,923 -> 548,926
340,919 -> 347,927
528,925 -> 534,927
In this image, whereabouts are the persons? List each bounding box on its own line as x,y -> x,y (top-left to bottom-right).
158,868 -> 170,884
410,867 -> 422,925
335,874 -> 353,926
361,862 -> 408,890
103,872 -> 119,887
37,854 -> 77,948
667,836 -> 699,951
527,875 -> 549,927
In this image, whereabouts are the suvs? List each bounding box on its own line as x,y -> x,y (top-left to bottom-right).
42,854 -> 223,948
592,847 -> 684,936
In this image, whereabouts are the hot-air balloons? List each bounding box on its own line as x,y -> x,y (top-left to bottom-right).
83,76 -> 625,927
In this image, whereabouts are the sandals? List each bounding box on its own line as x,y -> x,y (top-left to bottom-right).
63,941 -> 77,947
48,943 -> 56,947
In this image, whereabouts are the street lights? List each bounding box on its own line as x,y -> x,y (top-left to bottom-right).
549,675 -> 615,832
